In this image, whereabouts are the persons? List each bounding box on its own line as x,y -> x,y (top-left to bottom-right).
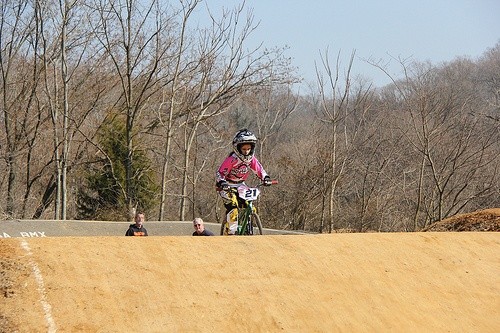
125,212 -> 148,236
215,128 -> 272,236
192,218 -> 215,236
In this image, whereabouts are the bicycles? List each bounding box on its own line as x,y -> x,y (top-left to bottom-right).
219,180 -> 279,236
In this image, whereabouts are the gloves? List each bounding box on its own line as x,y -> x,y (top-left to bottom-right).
264,176 -> 272,186
220,181 -> 230,192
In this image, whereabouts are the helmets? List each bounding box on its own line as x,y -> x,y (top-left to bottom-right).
232,128 -> 257,164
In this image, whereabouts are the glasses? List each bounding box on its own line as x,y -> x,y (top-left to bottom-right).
194,223 -> 201,226
